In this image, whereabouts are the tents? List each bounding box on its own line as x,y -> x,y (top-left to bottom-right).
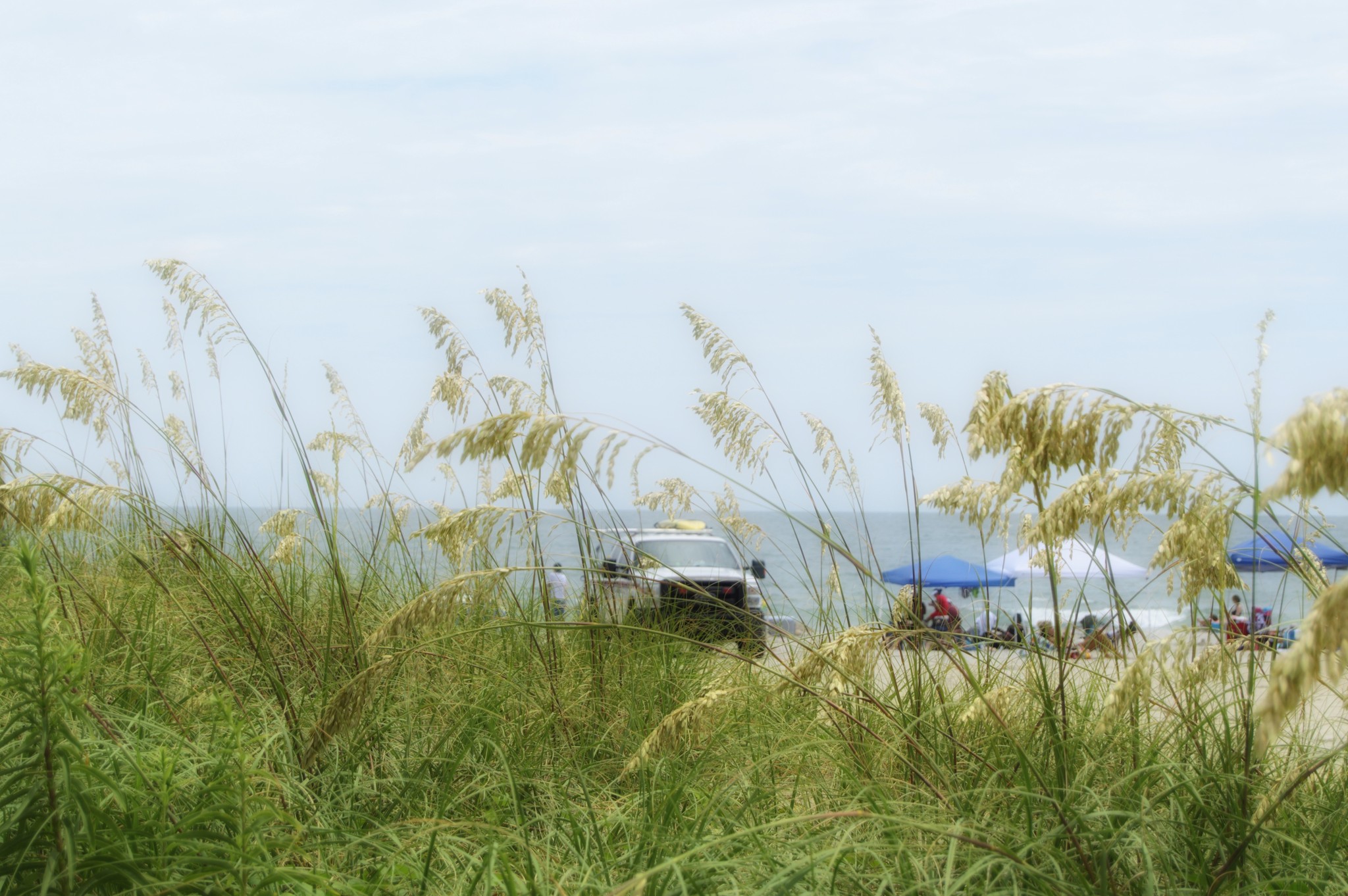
879,554 -> 1016,588
1222,529 -> 1348,572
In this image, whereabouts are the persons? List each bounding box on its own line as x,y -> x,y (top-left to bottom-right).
926,587 -> 988,651
1209,613 -> 1227,635
1007,613 -> 1026,649
904,590 -> 926,650
1229,593 -> 1242,622
547,562 -> 568,616
1037,620 -> 1127,659
1075,622 -> 1136,653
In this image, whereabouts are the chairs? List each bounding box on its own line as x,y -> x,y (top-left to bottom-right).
1226,606 -> 1274,641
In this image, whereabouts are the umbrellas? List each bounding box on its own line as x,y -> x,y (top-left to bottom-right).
986,536 -> 1146,578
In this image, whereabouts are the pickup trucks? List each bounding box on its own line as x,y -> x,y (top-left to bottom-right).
591,525 -> 770,658
